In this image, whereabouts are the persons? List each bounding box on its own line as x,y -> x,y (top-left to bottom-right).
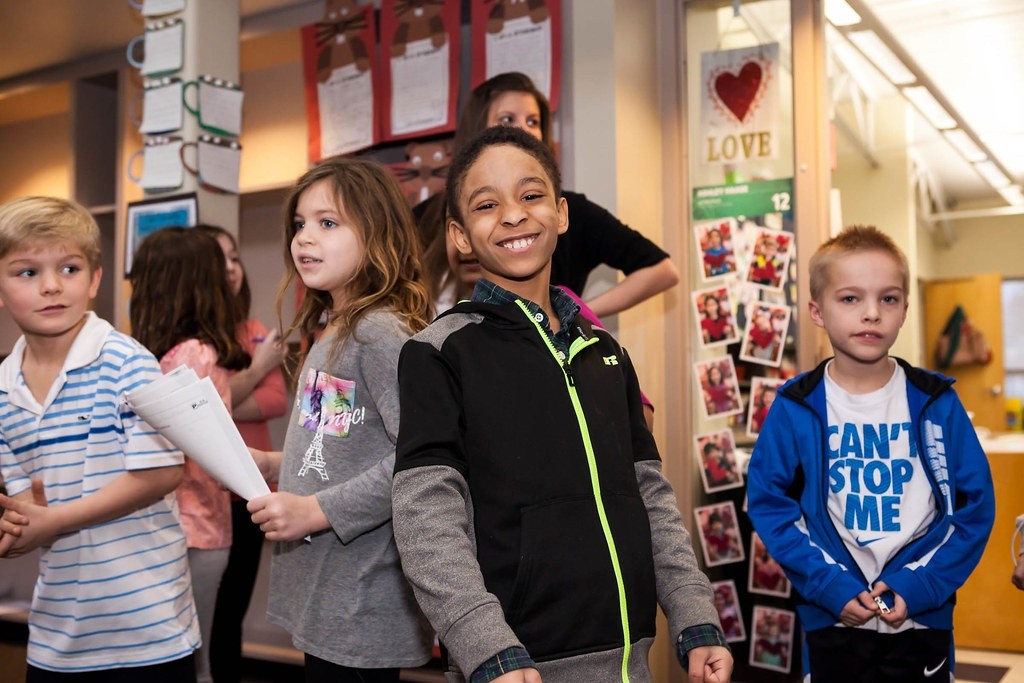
692,365 -> 791,675
703,229 -> 731,276
750,307 -> 787,361
208,156 -> 441,683
391,125 -> 734,683
746,224 -> 996,683
124,224 -> 288,683
701,295 -> 735,343
0,195 -> 187,683
749,234 -> 783,287
411,71 -> 681,322
